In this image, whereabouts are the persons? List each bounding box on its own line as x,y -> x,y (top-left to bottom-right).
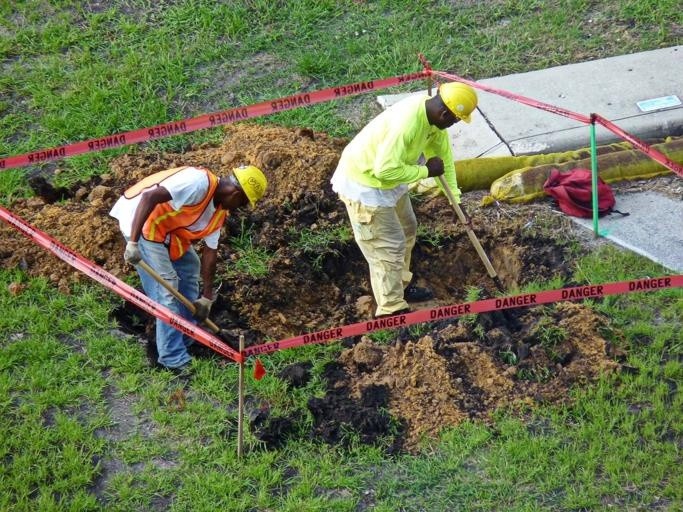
327,81 -> 477,322
106,164 -> 268,380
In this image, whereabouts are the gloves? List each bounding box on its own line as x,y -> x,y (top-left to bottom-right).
190,294 -> 216,326
122,239 -> 143,266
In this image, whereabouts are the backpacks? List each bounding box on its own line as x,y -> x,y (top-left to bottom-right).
543,167 -> 616,219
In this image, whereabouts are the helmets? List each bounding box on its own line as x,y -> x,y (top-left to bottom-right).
438,80 -> 479,124
231,164 -> 268,211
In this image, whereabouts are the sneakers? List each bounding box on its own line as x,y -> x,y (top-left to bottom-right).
165,358 -> 201,382
402,284 -> 434,303
374,307 -> 415,319
